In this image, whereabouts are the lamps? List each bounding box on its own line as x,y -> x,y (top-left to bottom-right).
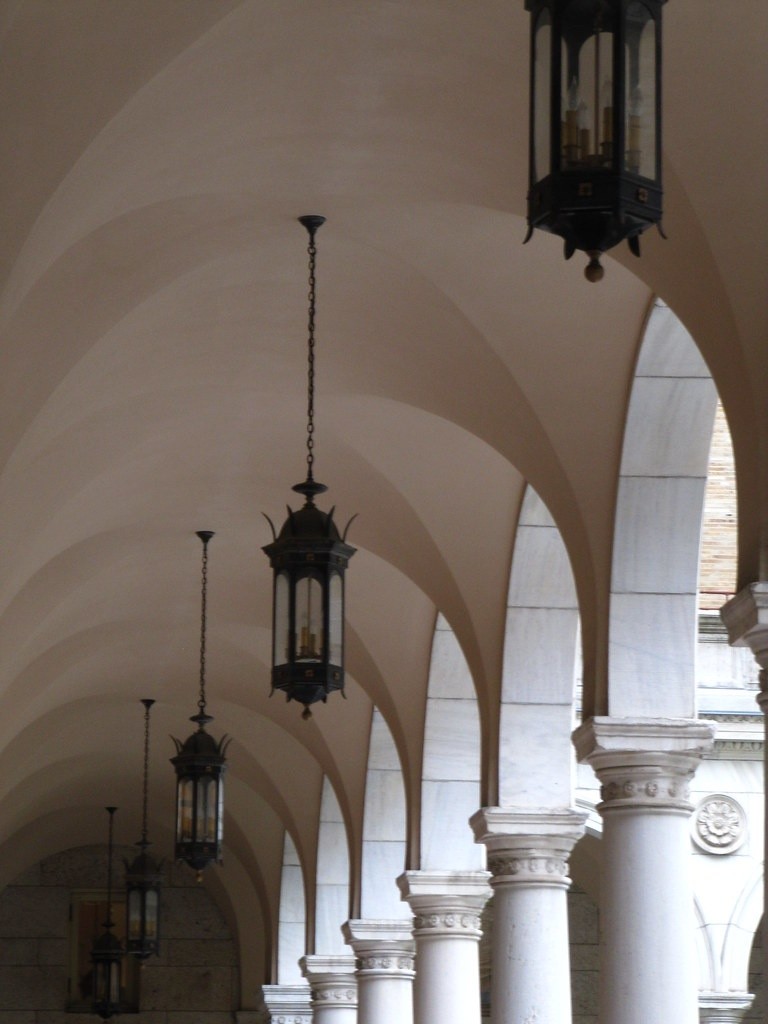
519,0 -> 671,284
168,530 -> 233,888
261,215 -> 361,720
87,805 -> 126,1024
125,699 -> 167,972
